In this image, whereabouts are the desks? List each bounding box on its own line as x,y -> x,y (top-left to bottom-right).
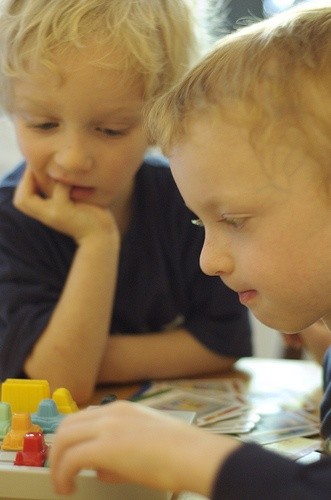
0,357 -> 322,500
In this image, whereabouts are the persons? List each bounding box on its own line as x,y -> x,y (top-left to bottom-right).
50,0 -> 331,500
1,0 -> 258,408
